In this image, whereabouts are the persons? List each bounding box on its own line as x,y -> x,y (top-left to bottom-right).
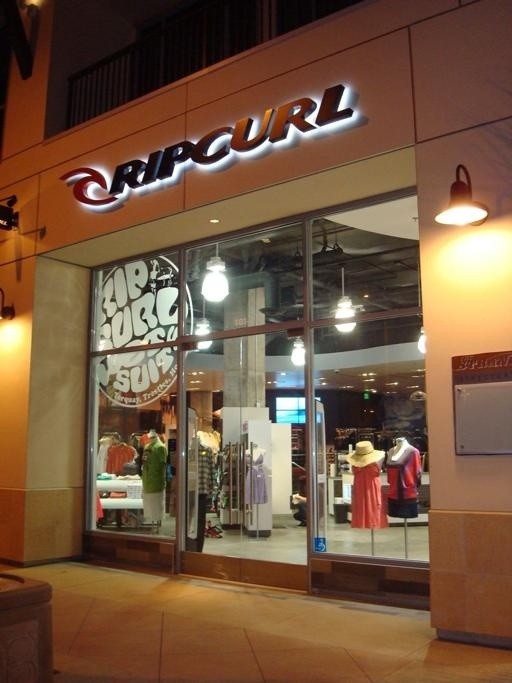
186,435 -> 215,557
384,435 -> 423,521
139,428 -> 166,447
292,493 -> 307,527
96,433 -> 114,474
243,442 -> 270,507
347,440 -> 388,535
137,431 -> 170,525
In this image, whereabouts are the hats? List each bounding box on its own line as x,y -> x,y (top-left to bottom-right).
345,441 -> 385,468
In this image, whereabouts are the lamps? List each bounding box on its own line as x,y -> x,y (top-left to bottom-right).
417,326 -> 426,354
291,317 -> 305,365
201,243 -> 229,302
195,296 -> 213,349
409,390 -> 426,401
434,164 -> 489,226
334,266 -> 357,332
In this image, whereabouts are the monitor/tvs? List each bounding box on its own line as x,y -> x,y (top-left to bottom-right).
271,393 -> 322,426
